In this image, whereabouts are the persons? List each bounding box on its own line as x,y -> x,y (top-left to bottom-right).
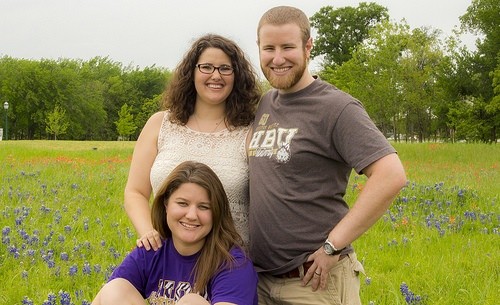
124,34 -> 262,253
246,6 -> 407,305
91,160 -> 259,305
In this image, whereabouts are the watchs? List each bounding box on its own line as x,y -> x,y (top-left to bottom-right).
323,236 -> 347,255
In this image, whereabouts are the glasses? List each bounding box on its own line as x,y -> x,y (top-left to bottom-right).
196,62 -> 234,76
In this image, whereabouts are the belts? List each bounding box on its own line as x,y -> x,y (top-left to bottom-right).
271,253 -> 348,279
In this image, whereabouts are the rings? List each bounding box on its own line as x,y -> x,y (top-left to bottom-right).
314,272 -> 321,276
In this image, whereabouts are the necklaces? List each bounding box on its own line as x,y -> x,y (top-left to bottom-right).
193,113 -> 224,139
